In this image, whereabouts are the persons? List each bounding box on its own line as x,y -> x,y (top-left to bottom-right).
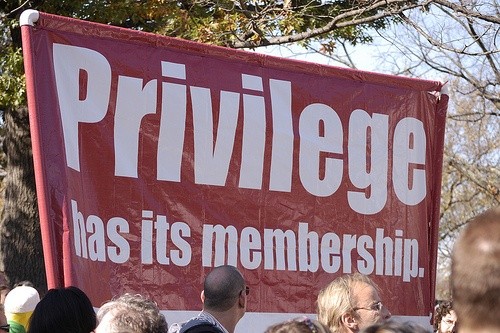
264,318 -> 331,333
176,265 -> 249,333
430,302 -> 459,333
30,286 -> 96,333
317,276 -> 392,333
448,206 -> 500,333
90,293 -> 168,333
4,285 -> 40,333
364,321 -> 429,333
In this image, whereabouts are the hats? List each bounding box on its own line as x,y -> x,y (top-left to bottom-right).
4,285 -> 40,314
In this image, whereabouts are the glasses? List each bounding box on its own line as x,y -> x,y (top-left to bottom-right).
351,301 -> 383,313
0,324 -> 10,332
293,315 -> 316,333
239,286 -> 249,295
